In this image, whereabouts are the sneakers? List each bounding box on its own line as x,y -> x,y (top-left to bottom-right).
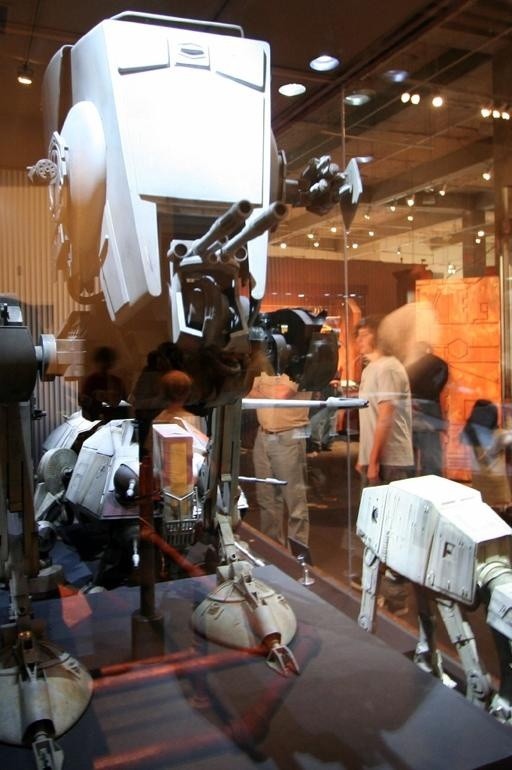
378,591 -> 409,616
351,575 -> 362,592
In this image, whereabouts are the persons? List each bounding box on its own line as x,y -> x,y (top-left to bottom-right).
351,314 -> 409,618
143,371 -> 204,454
249,371 -> 313,567
395,352 -> 448,477
78,345 -> 127,421
457,399 -> 512,512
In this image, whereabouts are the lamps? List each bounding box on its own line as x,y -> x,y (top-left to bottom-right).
493,103 -> 508,119
480,100 -> 494,117
381,55 -> 410,83
428,90 -> 444,107
280,241 -> 287,249
390,201 -> 396,212
278,78 -> 305,97
364,208 -> 371,219
396,247 -> 401,255
307,229 -> 317,239
501,107 -> 512,121
16,66 -> 35,86
313,240 -> 319,248
412,88 -> 429,105
438,185 -> 449,197
475,237 -> 482,244
482,167 -> 491,180
477,229 -> 484,237
308,46 -> 339,72
351,240 -> 358,248
401,89 -> 414,103
330,223 -> 336,233
344,83 -> 370,107
407,194 -> 415,206
369,229 -> 376,236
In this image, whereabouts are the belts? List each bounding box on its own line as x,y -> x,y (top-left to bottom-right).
258,424 -> 294,435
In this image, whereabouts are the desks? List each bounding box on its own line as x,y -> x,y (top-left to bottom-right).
0,564 -> 511,769
